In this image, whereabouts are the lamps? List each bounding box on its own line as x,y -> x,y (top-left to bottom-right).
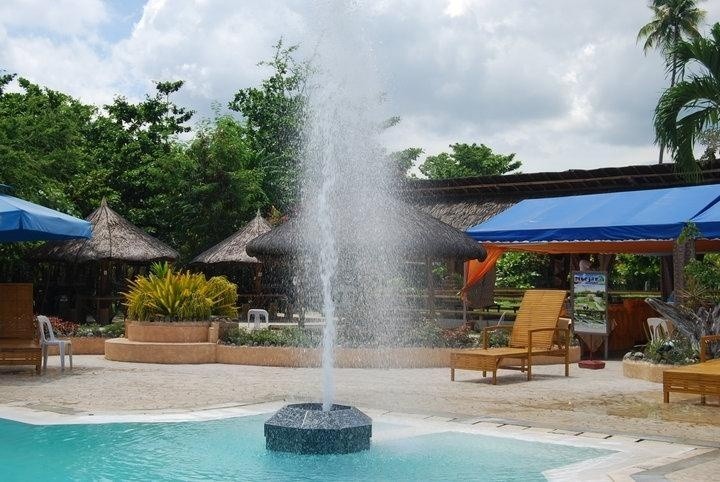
663,334 -> 719,406
37,315 -> 73,372
0,283 -> 41,375
450,289 -> 581,384
248,309 -> 269,330
647,318 -> 671,351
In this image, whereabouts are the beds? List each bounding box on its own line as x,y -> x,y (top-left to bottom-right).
0,193 -> 91,240
